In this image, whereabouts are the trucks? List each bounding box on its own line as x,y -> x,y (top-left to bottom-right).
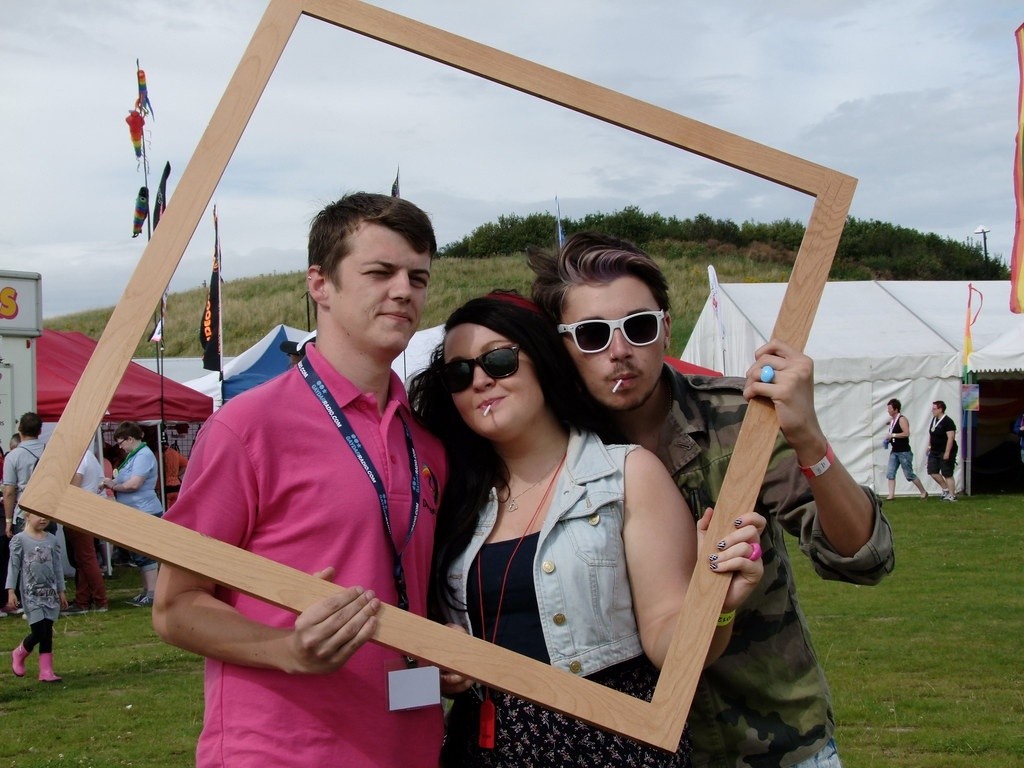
0,269 -> 43,456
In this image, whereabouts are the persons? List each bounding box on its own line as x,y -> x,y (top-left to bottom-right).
153,193 -> 444,767
882,399 -> 929,501
406,231 -> 894,768
0,413 -> 114,614
103,420 -> 164,608
6,511 -> 71,682
926,400 -> 959,502
1015,412 -> 1023,471
164,447 -> 187,510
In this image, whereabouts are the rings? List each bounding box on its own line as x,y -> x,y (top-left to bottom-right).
758,364 -> 775,383
749,542 -> 762,561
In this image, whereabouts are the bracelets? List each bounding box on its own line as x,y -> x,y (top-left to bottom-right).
111,483 -> 118,490
6,518 -> 11,523
718,609 -> 737,626
794,442 -> 836,477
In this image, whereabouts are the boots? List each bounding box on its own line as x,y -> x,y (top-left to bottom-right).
39,653 -> 62,681
12,641 -> 30,676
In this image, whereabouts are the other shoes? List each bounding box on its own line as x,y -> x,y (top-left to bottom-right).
100,566 -> 112,572
0,602 -> 24,614
921,492 -> 928,499
943,495 -> 957,501
59,602 -> 89,616
129,560 -> 138,567
940,491 -> 949,499
114,557 -> 128,565
0,611 -> 8,617
886,498 -> 895,501
89,603 -> 108,612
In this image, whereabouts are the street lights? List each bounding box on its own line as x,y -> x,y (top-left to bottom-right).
974,225 -> 990,280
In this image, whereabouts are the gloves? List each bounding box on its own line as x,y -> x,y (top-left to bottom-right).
882,440 -> 889,449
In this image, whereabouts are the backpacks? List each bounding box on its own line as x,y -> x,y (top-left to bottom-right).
21,444 -> 46,471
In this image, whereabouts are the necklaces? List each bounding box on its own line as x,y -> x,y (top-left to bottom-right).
503,451 -> 562,513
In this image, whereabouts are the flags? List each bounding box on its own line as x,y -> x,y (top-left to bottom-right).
149,161 -> 174,342
199,206 -> 225,372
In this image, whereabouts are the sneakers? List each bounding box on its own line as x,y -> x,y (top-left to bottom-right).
133,596 -> 153,607
125,593 -> 145,604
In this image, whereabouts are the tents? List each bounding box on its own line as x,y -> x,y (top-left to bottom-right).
33,328 -> 214,575
678,265 -> 1024,496
216,319 -> 445,397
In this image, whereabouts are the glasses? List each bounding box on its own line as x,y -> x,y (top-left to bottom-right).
118,439 -> 126,445
559,309 -> 666,353
437,344 -> 523,393
